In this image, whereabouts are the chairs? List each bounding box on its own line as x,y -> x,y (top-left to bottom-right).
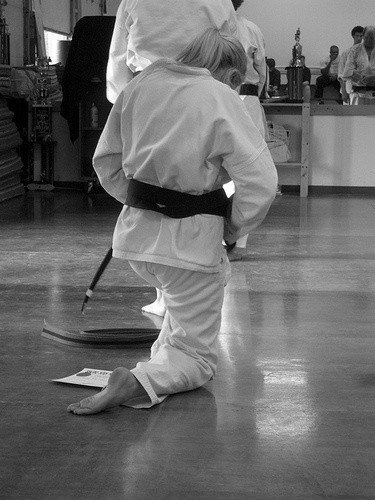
312,76 -> 375,106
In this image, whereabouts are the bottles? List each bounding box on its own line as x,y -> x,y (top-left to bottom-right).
90,102 -> 99,127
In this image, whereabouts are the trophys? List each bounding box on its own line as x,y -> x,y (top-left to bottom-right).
285,27 -> 306,103
28,56 -> 53,139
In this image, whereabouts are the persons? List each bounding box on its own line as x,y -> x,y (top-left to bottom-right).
300,56 -> 311,86
106,0 -> 239,105
265,56 -> 280,98
231,0 -> 265,140
315,46 -> 343,104
67,26 -> 278,416
337,26 -> 364,105
341,26 -> 375,105
